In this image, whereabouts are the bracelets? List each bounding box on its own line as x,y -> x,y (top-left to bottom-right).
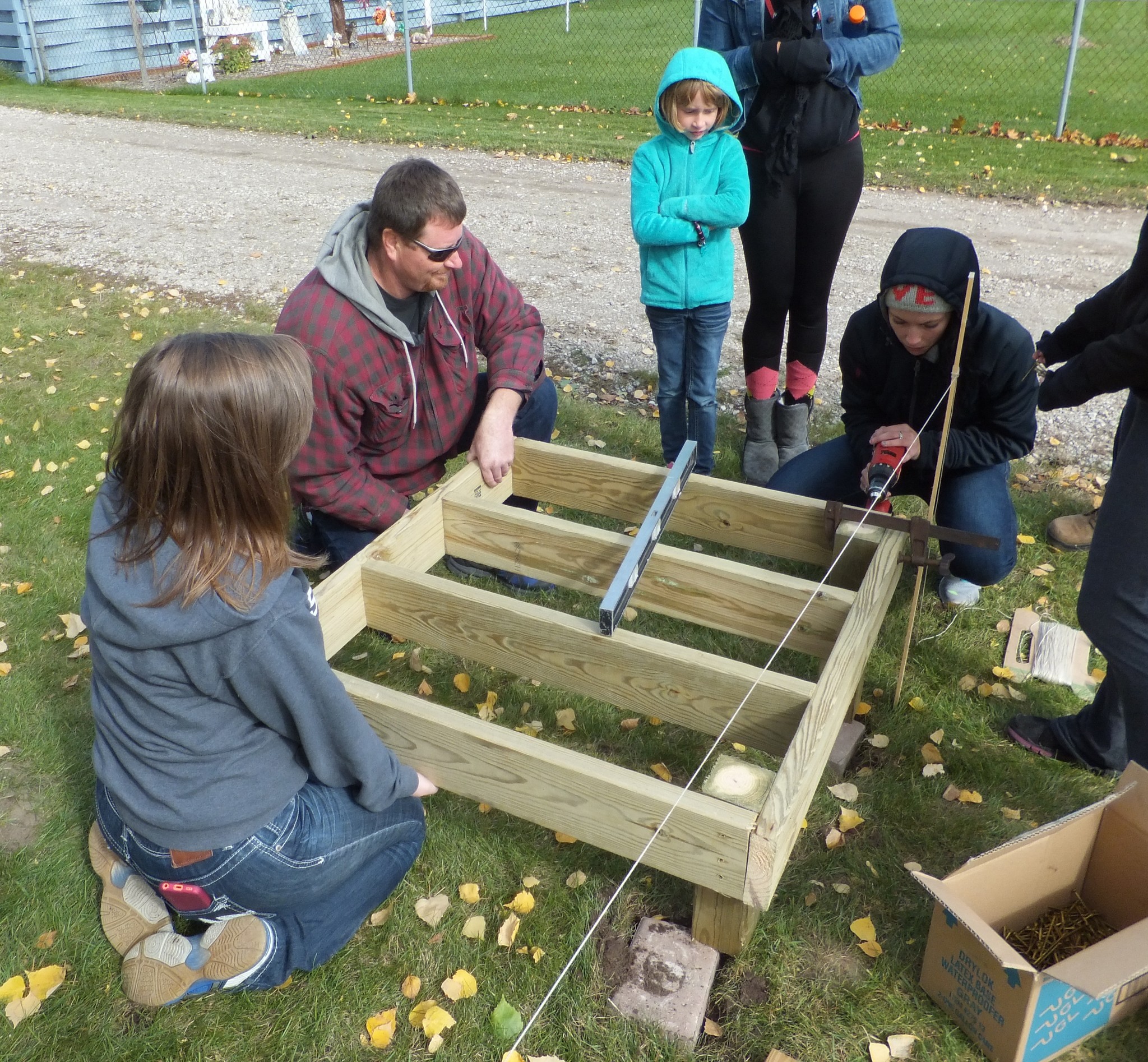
692,220 -> 706,248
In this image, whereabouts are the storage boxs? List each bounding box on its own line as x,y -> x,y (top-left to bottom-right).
908,758 -> 1148,1062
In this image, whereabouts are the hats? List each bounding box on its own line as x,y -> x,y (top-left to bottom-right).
885,284 -> 951,313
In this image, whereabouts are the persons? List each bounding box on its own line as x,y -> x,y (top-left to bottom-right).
273,157 -> 572,593
765,228 -> 1041,609
1007,209 -> 1147,790
629,46 -> 750,477
700,1 -> 902,487
88,336 -> 439,1004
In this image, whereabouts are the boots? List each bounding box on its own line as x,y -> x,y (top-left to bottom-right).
776,391 -> 814,469
743,388 -> 779,486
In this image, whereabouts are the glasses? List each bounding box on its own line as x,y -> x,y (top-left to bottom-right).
398,230 -> 464,262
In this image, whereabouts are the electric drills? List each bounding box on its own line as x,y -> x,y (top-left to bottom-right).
867,440 -> 907,513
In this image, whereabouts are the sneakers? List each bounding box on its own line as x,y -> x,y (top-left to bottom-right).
121,915 -> 274,1008
88,819 -> 174,958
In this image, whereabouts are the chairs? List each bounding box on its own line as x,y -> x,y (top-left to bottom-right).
199,0 -> 271,64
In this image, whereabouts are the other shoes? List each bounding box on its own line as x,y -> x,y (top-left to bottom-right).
939,573 -> 981,606
446,555 -> 556,595
293,502 -> 319,556
1008,716 -> 1078,765
